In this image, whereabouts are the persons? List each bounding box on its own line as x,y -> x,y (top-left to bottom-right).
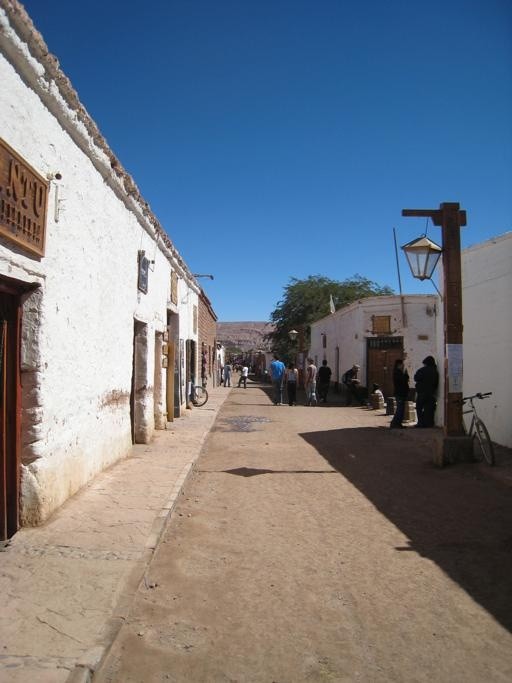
269,353 -> 287,406
223,361 -> 231,387
231,364 -> 252,372
343,363 -> 366,405
286,361 -> 299,407
373,383 -> 384,408
390,358 -> 410,429
317,359 -> 332,403
236,362 -> 249,388
413,355 -> 439,427
305,357 -> 317,406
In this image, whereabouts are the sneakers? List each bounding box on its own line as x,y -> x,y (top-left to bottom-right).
273,399 -> 328,406
390,421 -> 434,429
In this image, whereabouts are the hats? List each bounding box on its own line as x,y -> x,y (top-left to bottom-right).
422,356 -> 435,366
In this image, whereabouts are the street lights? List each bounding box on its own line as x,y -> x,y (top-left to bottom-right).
287,329 -> 299,348
399,216 -> 442,299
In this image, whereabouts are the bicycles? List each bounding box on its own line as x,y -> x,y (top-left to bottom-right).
452,392 -> 495,466
190,383 -> 208,407
